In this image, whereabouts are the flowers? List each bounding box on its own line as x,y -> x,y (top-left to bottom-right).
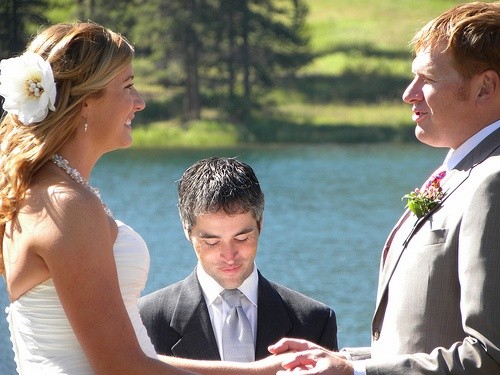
400,170 -> 450,246
0,53 -> 57,125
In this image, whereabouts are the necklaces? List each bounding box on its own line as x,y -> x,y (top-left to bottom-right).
51,153 -> 112,216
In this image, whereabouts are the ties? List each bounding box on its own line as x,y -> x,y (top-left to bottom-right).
220,287 -> 255,363
425,169 -> 446,188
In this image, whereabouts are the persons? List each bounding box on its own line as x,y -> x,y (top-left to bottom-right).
138,157 -> 338,362
268,2 -> 500,375
0,23 -> 298,375
